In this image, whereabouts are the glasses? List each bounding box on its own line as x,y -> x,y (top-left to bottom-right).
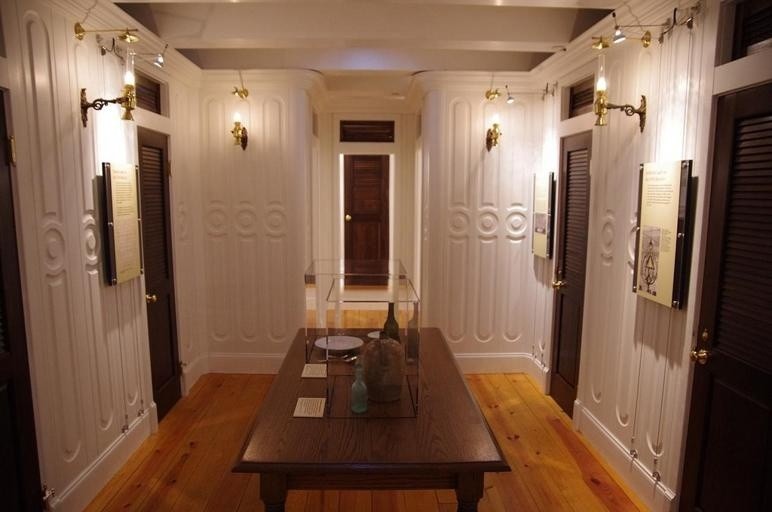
594,53 -> 645,132
486,82 -> 514,104
99,38 -> 168,70
74,22 -> 139,57
591,32 -> 653,50
614,8 -> 693,43
486,114 -> 500,151
232,74 -> 249,98
232,110 -> 247,149
81,68 -> 137,126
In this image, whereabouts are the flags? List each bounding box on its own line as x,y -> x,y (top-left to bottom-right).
384,301 -> 399,341
407,302 -> 419,358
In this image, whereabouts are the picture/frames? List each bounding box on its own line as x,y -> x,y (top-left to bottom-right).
229,273 -> 511,512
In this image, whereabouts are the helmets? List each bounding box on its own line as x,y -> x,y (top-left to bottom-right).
315,335 -> 364,351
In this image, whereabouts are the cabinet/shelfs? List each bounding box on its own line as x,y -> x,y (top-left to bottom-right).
407,301 -> 418,359
383,302 -> 399,341
350,364 -> 369,414
